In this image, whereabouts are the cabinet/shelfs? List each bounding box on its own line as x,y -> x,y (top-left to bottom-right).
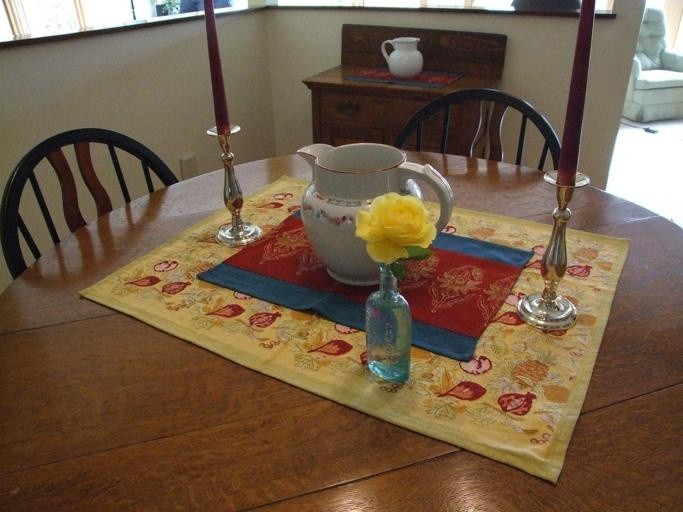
304,60 -> 487,157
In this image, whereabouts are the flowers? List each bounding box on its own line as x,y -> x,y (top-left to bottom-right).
353,191 -> 438,277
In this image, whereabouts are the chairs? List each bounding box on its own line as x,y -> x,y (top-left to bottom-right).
393,86 -> 560,174
1,125 -> 178,278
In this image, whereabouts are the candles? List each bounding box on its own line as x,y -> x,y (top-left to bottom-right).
556,0 -> 594,185
204,0 -> 231,133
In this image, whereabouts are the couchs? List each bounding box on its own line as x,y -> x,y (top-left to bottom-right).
621,5 -> 683,124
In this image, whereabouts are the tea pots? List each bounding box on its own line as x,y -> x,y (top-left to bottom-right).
296,143 -> 455,288
379,37 -> 424,78
